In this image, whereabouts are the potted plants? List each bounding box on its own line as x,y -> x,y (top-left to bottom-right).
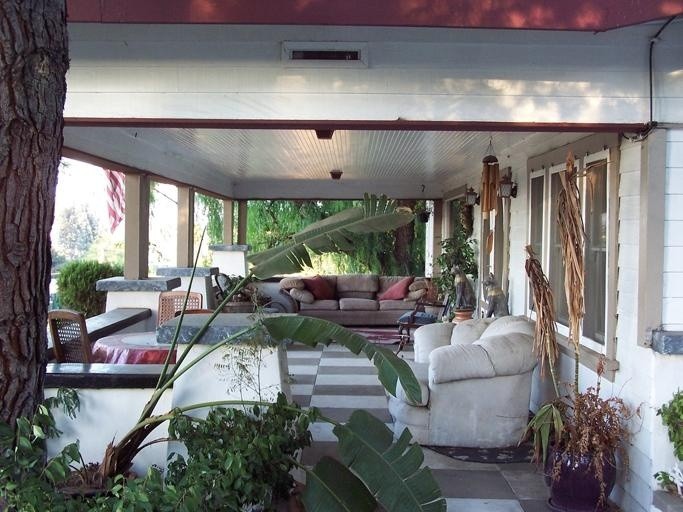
509,153 -> 639,512
415,202 -> 431,222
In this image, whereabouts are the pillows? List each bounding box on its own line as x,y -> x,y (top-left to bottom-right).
378,275 -> 416,300
303,273 -> 335,299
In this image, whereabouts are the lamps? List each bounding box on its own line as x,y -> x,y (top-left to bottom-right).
464,184 -> 481,207
497,175 -> 518,200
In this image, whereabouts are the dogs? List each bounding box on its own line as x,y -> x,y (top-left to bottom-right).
481,273 -> 509,317
449,264 -> 476,309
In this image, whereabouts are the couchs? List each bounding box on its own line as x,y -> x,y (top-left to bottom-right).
270,274 -> 431,328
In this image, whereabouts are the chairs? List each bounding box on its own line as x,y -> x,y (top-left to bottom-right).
214,273 -> 243,301
383,294 -> 540,456
47,288 -> 203,368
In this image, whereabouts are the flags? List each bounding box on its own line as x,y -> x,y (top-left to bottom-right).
104,169 -> 125,233
